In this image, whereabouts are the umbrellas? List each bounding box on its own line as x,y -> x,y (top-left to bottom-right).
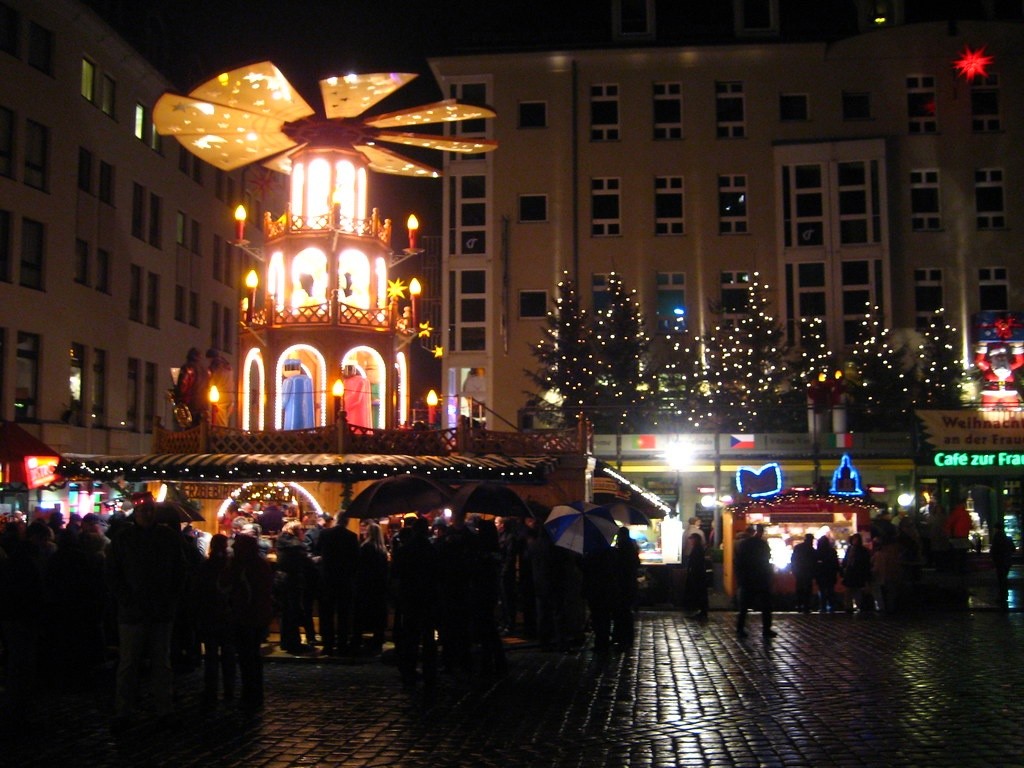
132,474 -> 650,557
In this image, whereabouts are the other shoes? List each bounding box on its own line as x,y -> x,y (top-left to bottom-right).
10,597 -> 891,741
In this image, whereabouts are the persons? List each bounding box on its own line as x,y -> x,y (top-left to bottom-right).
975,342 -> 1024,411
170,346 -> 234,428
0,499 -> 1017,727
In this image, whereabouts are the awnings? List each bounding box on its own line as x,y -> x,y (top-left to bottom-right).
0,421 -> 62,490
